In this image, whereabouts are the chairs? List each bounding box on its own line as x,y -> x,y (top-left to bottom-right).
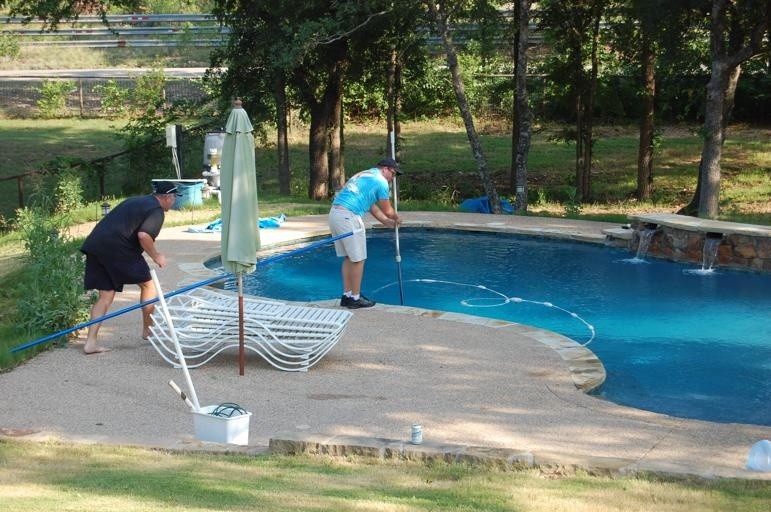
146,287 -> 353,372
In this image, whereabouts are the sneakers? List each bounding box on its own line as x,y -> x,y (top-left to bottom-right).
348,294 -> 376,309
340,294 -> 352,307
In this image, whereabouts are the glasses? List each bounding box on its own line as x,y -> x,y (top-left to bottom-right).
388,168 -> 396,177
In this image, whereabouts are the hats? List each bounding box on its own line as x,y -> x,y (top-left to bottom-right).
156,182 -> 183,197
377,158 -> 403,176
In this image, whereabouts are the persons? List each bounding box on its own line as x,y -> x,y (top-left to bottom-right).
327,154 -> 402,308
78,179 -> 176,354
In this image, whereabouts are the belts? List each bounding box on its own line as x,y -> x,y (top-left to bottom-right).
332,205 -> 346,210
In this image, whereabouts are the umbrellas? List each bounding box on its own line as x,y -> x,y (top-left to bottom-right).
216,97 -> 266,376
